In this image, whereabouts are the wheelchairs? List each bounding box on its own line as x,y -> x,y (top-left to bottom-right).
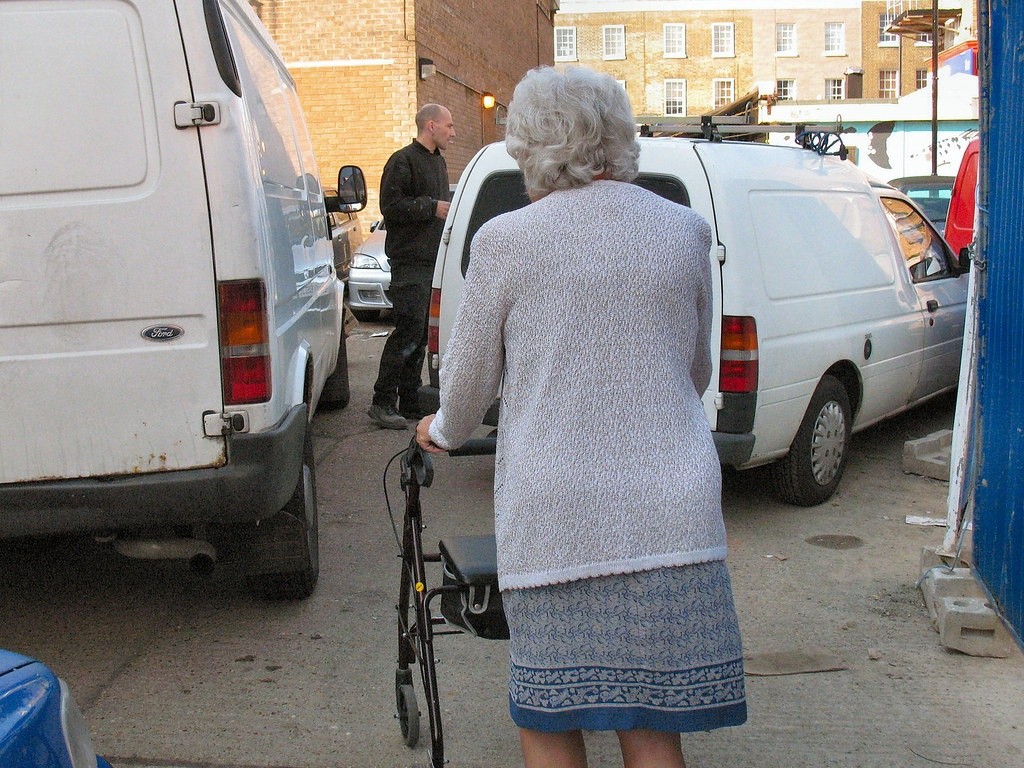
384,419 -> 510,768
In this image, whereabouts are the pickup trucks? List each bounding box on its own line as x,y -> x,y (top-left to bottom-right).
417,86 -> 976,507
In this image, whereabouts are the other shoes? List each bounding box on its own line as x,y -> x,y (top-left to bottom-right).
368,403 -> 408,429
399,406 -> 430,419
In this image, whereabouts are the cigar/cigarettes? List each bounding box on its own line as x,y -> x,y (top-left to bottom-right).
448,140 -> 454,144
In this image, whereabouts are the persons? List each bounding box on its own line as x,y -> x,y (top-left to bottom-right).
367,102 -> 456,431
417,64 -> 747,768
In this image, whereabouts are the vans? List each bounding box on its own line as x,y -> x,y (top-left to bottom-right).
940,138 -> 980,256
1,0 -> 368,599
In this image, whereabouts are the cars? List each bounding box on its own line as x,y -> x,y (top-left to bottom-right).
346,184 -> 460,324
887,174 -> 957,231
322,186 -> 363,293
